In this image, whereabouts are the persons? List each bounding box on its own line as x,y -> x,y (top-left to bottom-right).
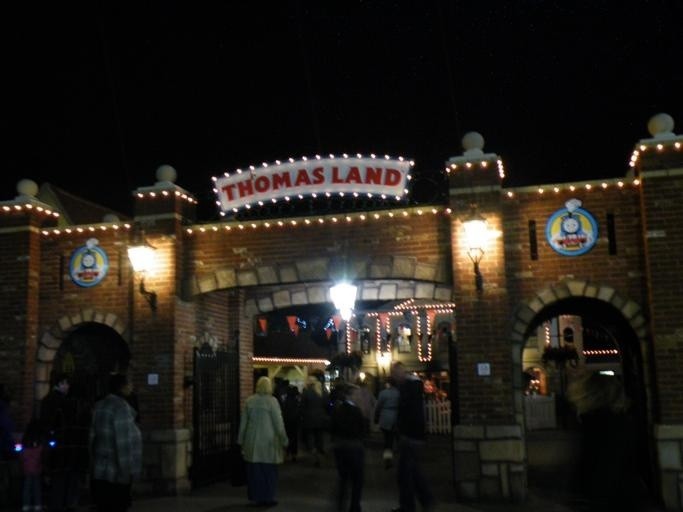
0,371 -> 144,512
563,373 -> 643,512
237,361 -> 437,512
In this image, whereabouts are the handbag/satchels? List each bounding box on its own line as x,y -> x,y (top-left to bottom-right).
229,454 -> 246,487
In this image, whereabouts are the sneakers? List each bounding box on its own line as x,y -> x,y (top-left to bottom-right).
23,505 -> 42,511
